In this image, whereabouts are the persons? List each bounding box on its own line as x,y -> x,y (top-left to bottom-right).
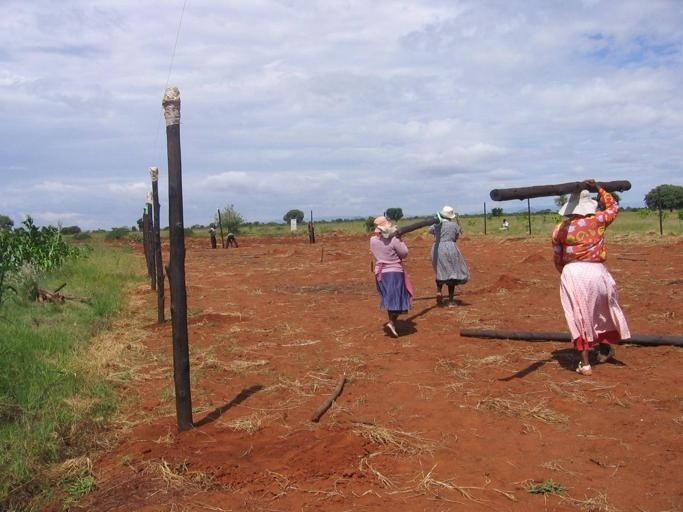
308,223 -> 315,244
208,223 -> 217,248
501,218 -> 509,230
225,232 -> 238,248
427,206 -> 471,308
368,216 -> 415,338
551,179 -> 632,376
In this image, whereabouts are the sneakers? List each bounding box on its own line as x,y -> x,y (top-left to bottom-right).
447,301 -> 458,307
576,361 -> 593,376
596,346 -> 616,364
436,292 -> 443,302
386,323 -> 399,338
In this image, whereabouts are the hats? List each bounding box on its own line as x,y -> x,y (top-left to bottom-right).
438,205 -> 456,219
558,189 -> 598,216
373,216 -> 392,232
